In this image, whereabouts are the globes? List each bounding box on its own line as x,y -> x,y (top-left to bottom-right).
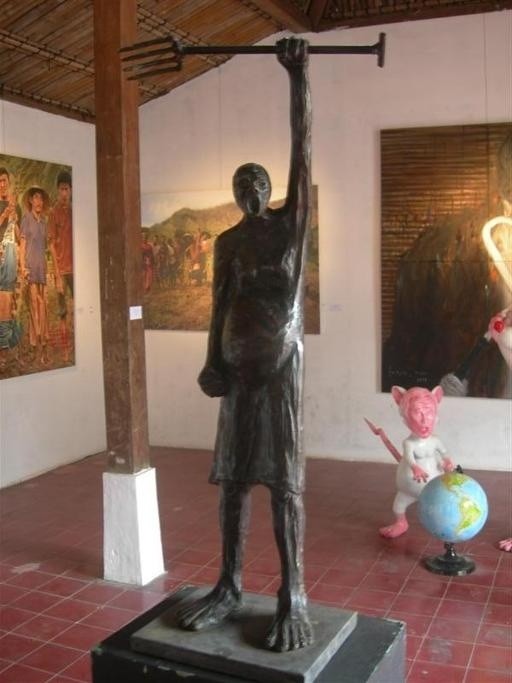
417,472 -> 488,575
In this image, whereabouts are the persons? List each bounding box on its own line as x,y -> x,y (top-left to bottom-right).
1,166 -> 24,347
18,182 -> 53,350
142,228 -> 213,296
171,35 -> 314,651
45,167 -> 74,341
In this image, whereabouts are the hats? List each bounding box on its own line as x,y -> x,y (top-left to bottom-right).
22,187 -> 49,211
